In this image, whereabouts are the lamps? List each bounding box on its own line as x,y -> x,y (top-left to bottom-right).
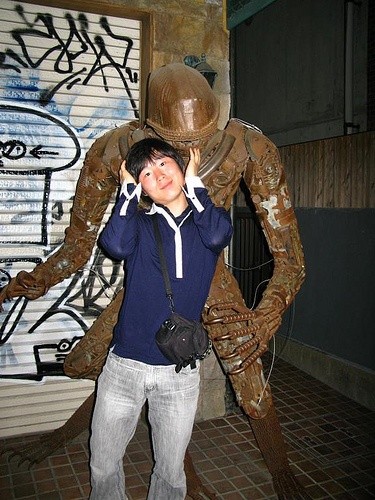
183,52 -> 217,90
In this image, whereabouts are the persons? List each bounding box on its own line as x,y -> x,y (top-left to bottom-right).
90,137 -> 233,499
0,64 -> 312,499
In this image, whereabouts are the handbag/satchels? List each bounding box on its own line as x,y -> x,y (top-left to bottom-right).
154,311 -> 211,371
